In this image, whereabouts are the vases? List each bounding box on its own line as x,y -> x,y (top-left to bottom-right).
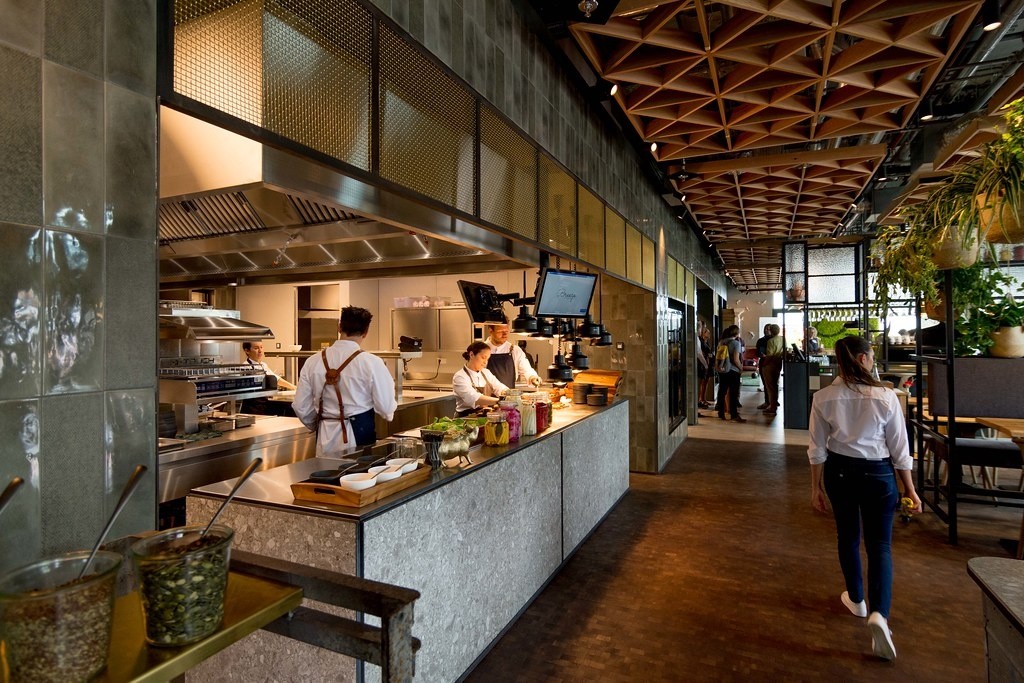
903,388 -> 911,398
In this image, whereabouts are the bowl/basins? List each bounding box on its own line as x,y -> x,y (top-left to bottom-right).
0,550 -> 122,683
386,458 -> 418,475
339,473 -> 377,491
338,463 -> 371,475
368,466 -> 403,485
130,522 -> 234,648
289,345 -> 302,352
356,455 -> 386,472
310,470 -> 345,495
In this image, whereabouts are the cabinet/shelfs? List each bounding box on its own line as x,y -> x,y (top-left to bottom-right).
388,302 -> 468,388
910,259 -> 1024,546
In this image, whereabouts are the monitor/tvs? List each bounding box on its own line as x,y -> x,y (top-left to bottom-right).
456,280 -> 508,324
791,343 -> 805,360
533,267 -> 598,318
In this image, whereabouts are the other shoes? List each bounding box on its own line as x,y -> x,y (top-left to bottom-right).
757,403 -> 769,410
699,401 -> 711,409
698,413 -> 702,417
763,409 -> 776,415
731,417 -> 747,423
718,412 -> 726,420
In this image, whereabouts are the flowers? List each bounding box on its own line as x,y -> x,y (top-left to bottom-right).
903,381 -> 914,388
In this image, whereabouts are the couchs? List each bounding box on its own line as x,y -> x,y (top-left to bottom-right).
742,348 -> 759,370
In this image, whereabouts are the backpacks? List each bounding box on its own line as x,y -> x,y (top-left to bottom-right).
715,339 -> 734,374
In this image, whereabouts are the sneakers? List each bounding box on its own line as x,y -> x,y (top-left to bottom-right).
841,591 -> 867,618
867,612 -> 896,660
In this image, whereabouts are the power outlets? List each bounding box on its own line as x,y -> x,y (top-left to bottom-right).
437,358 -> 446,364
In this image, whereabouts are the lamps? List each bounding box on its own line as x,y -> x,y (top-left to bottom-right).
509,270 -> 538,334
673,191 -> 686,202
878,165 -> 887,180
677,209 -> 687,219
839,215 -> 849,227
919,99 -> 933,120
527,270 -> 554,340
832,231 -> 838,239
597,78 -> 618,95
544,200 -> 575,385
697,227 -> 706,235
852,195 -> 864,208
705,240 -> 712,248
982,0 -> 1002,31
548,198 -> 565,337
589,224 -> 612,348
564,341 -> 590,372
559,224 -> 583,342
577,214 -> 601,340
641,142 -> 657,151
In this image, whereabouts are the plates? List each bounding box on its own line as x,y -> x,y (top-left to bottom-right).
573,384 -> 608,406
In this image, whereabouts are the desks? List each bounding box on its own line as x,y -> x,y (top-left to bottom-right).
922,410 -> 999,507
975,417 -> 1024,560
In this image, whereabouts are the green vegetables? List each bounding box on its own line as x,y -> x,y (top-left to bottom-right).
141,534 -> 229,645
421,415 -> 487,431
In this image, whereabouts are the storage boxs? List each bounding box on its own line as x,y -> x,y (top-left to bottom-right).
198,417 -> 235,432
221,414 -> 256,428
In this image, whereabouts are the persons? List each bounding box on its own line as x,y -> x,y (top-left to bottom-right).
291,305 -> 397,459
240,342 -> 297,415
697,320 -> 825,422
453,342 -> 510,418
484,314 -> 543,389
807,335 -> 922,660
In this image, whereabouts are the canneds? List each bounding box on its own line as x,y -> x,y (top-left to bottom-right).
484,388 -> 553,446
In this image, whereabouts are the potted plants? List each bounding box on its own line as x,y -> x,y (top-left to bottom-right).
857,96 -> 1024,360
